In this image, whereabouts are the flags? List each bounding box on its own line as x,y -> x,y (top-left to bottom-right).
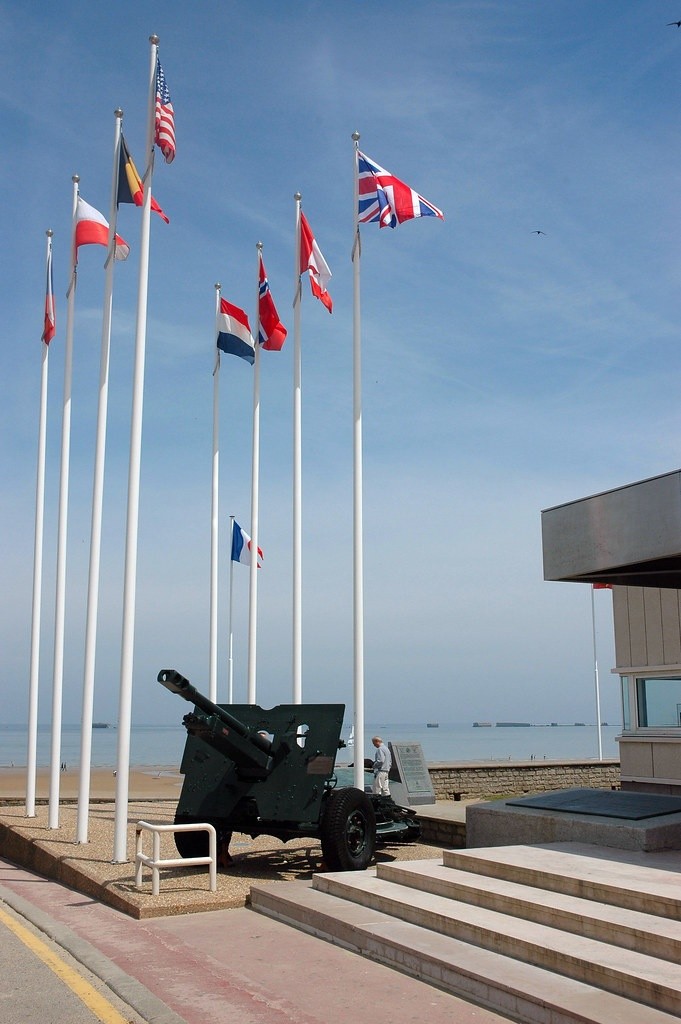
216,297 -> 256,366
40,244 -> 57,349
230,520 -> 264,569
153,48 -> 176,165
299,209 -> 334,314
357,150 -> 445,232
74,193 -> 130,273
258,252 -> 288,353
116,133 -> 172,225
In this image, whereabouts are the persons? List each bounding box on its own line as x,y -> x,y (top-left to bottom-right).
371,736 -> 393,796
529,753 -> 546,762
60,761 -> 67,771
